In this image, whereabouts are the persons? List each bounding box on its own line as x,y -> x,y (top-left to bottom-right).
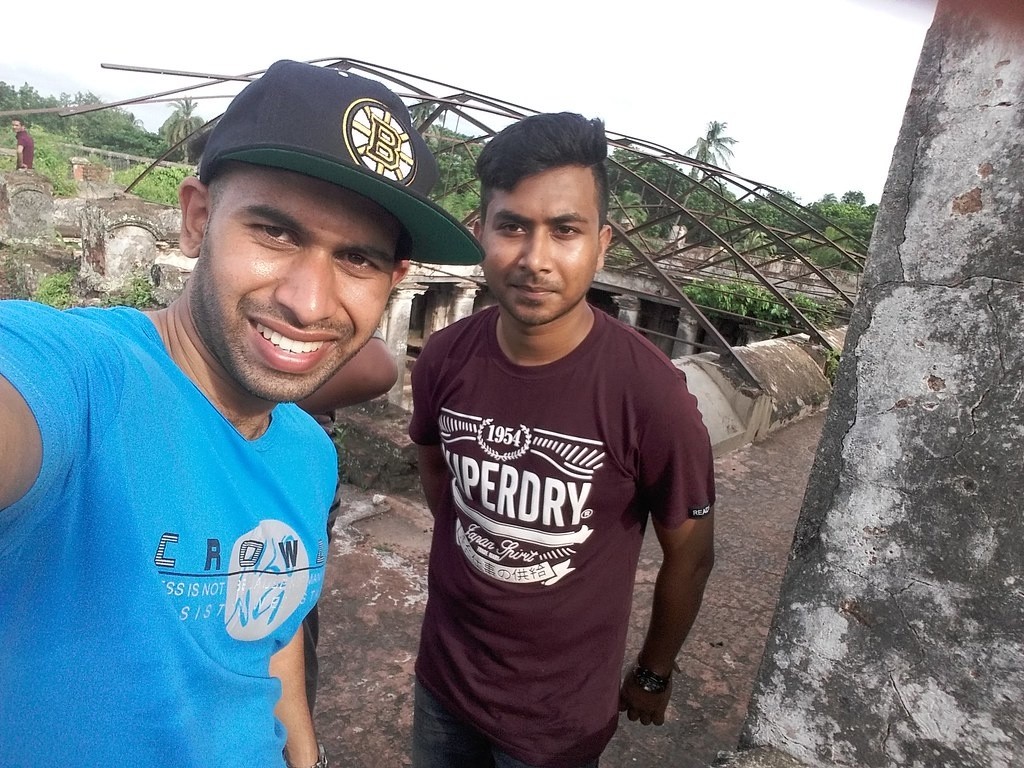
1,61 -> 489,767
12,117 -> 34,173
407,113 -> 720,768
291,328 -> 399,718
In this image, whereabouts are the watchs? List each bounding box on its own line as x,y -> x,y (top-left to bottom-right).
633,651 -> 674,694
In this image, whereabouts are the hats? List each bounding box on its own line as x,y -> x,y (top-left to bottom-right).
200,60 -> 486,265
